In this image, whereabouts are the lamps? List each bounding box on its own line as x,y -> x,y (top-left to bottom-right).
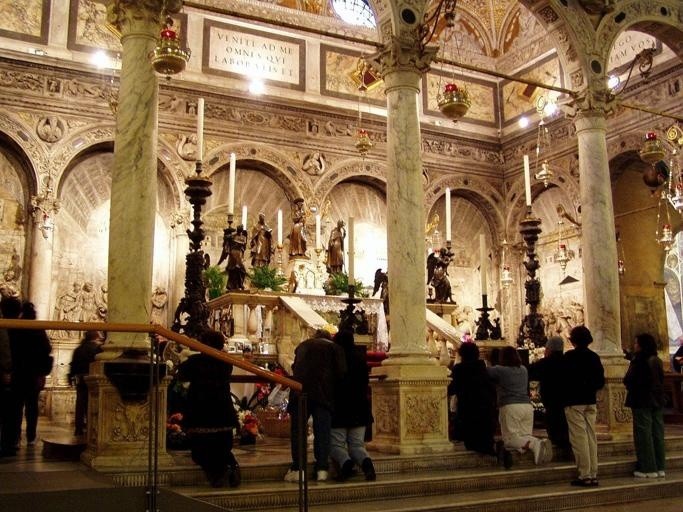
330,0 -> 376,30
605,75 -> 622,93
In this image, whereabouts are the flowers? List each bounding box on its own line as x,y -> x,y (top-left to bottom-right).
240,408 -> 262,438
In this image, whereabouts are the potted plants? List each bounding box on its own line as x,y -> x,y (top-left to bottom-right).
202,265 -> 226,300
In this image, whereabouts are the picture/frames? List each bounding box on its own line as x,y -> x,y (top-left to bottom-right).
422,68 -> 497,128
67,0 -> 187,65
202,18 -> 306,91
499,53 -> 565,128
603,39 -> 662,77
320,44 -> 387,108
0,1 -> 51,44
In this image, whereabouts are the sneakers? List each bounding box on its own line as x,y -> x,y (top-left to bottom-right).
284,468 -> 304,482
531,441 -> 543,465
363,457 -> 375,480
571,479 -> 598,486
335,459 -> 354,481
317,470 -> 328,481
634,471 -> 665,478
542,438 -> 553,463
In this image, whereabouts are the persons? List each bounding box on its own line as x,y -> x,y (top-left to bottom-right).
544,298 -> 583,337
432,247 -> 455,305
288,201 -> 306,257
623,331 -> 682,479
173,328 -> 243,489
151,284 -> 168,325
326,219 -> 347,274
0,296 -> 55,458
447,325 -> 605,488
225,224 -> 247,287
0,270 -> 20,298
68,330 -> 102,438
55,280 -> 108,337
251,213 -> 272,267
456,304 -> 477,337
328,330 -> 376,482
284,329 -> 347,483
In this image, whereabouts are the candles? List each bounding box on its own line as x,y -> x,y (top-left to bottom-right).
278,209 -> 283,246
316,216 -> 321,249
348,217 -> 355,284
228,152 -> 236,212
479,233 -> 487,294
445,188 -> 451,242
524,156 -> 532,206
242,206 -> 248,229
197,97 -> 204,159
